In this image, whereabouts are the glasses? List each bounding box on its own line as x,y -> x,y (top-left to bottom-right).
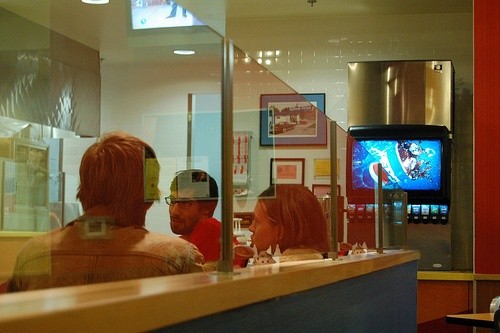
165,195 -> 200,210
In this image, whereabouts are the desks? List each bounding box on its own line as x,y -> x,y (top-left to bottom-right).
445,312 -> 495,328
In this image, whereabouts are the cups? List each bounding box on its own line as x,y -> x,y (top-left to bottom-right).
232,245 -> 255,269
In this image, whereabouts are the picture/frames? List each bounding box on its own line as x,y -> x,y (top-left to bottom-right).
270,158 -> 304,186
312,184 -> 341,197
260,93 -> 327,145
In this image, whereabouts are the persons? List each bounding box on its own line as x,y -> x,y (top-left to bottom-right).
164,169 -> 241,268
4,132 -> 206,293
249,184 -> 330,264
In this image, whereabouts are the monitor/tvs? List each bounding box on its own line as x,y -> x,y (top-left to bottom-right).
125,0 -> 222,48
343,123 -> 452,206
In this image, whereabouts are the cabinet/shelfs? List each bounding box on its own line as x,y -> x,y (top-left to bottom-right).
0,137 -> 49,209
